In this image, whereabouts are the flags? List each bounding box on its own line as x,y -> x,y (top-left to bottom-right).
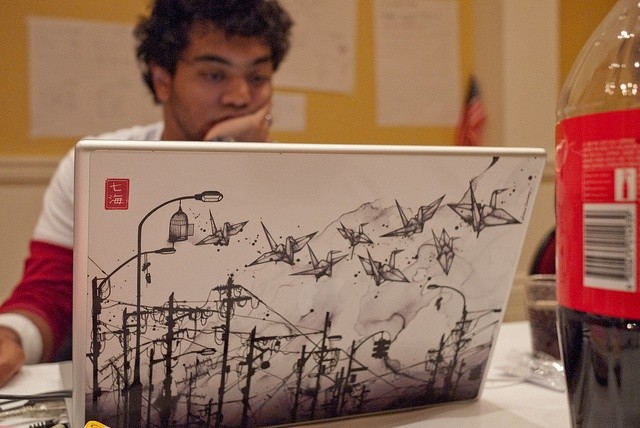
454,74 -> 487,147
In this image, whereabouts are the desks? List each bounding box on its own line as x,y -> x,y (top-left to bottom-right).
0,320 -> 571,427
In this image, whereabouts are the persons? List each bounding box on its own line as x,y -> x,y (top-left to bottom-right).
0,1 -> 294,391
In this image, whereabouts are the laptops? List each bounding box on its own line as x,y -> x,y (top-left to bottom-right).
63,140 -> 548,427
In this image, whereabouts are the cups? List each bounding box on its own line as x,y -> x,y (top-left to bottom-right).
522,274 -> 562,361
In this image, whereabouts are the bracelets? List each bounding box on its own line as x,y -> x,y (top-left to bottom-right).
0,312 -> 44,366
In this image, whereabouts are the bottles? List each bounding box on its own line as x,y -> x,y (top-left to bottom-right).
553,1 -> 640,427
